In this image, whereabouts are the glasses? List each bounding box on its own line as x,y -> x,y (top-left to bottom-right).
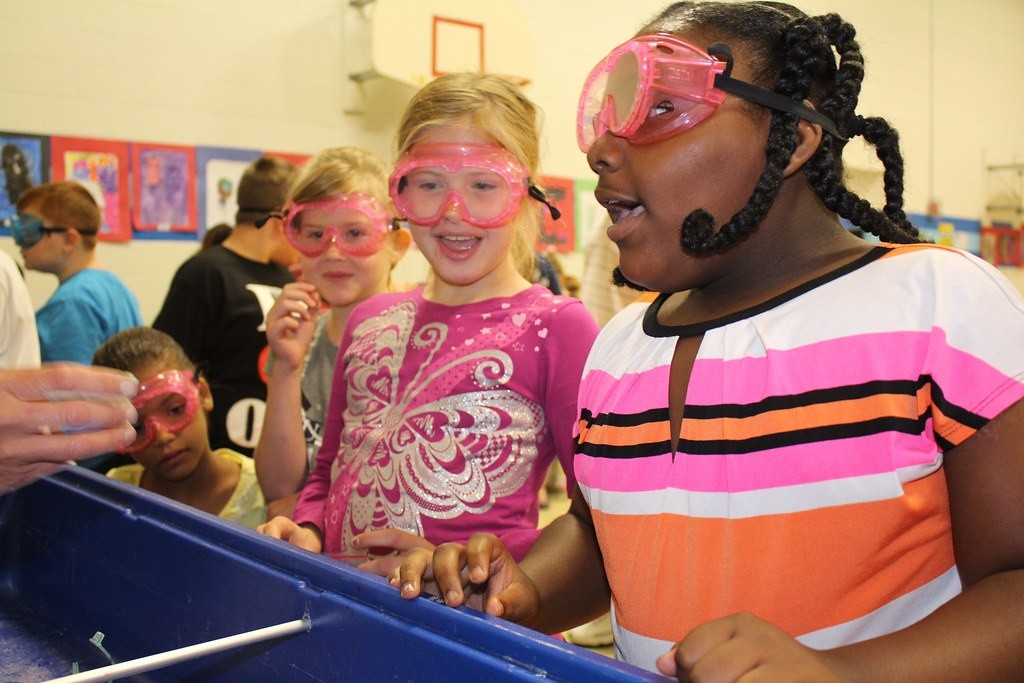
389,143 -> 562,229
11,214 -> 97,247
256,194 -> 408,257
577,33 -> 851,155
118,366 -> 201,453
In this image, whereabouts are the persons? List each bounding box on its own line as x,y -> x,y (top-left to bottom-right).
0,0 -> 1024,683
